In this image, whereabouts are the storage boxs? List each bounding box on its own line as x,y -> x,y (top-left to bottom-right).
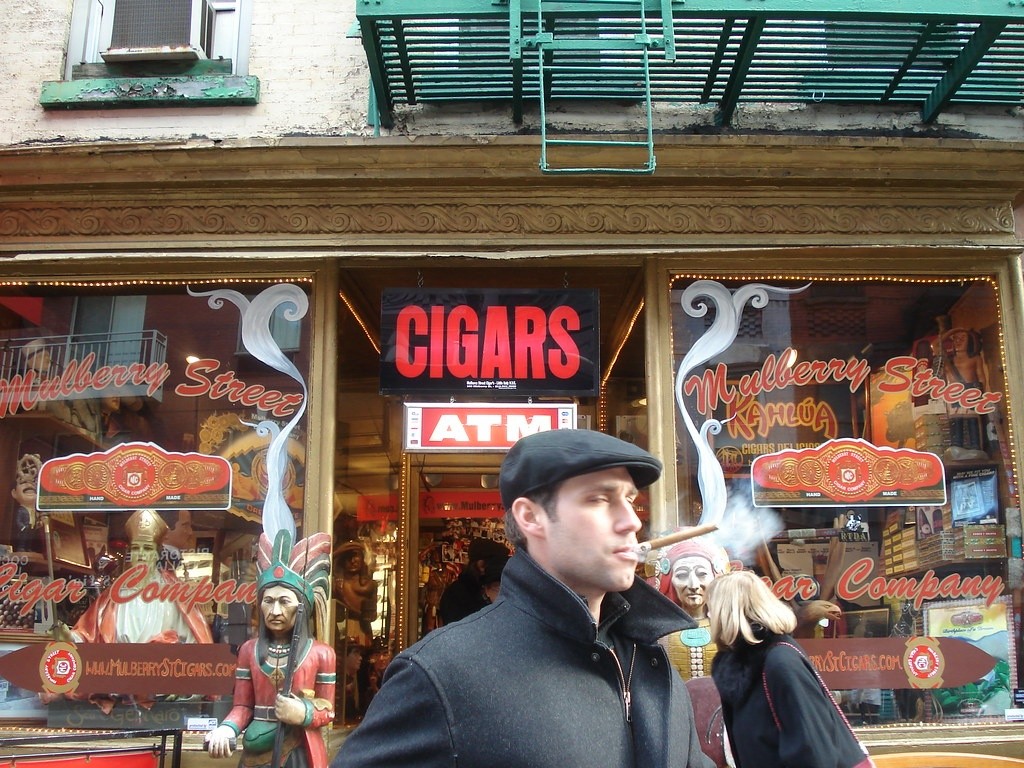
777,411 -> 1008,607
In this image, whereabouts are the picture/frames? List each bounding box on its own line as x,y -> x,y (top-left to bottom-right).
41,512 -> 92,571
923,595 -> 1019,725
829,607 -> 891,718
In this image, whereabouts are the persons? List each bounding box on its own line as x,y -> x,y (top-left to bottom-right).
931,326 -> 987,449
437,535 -> 510,629
330,539 -> 378,648
19,340 -> 194,453
10,474 -> 44,551
850,650 -> 881,723
644,526 -> 731,768
326,429 -> 717,768
203,528 -> 337,768
702,571 -> 870,768
344,635 -> 388,723
158,509 -> 193,571
39,509 -> 219,699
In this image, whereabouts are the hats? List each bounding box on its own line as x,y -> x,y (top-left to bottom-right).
479,561 -> 505,581
468,538 -> 510,558
499,429 -> 662,512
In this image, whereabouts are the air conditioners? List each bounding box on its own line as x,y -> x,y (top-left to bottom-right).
98,0 -> 218,62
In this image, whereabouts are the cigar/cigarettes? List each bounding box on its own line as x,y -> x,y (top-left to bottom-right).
632,523 -> 718,553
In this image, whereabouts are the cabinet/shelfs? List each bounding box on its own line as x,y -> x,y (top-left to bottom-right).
881,458 -> 1012,581
